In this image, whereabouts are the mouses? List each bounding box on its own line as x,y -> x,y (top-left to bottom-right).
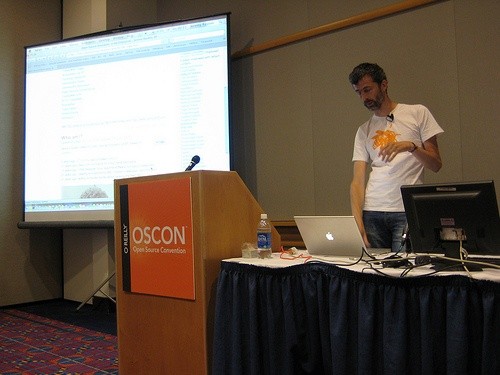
415,255 -> 431,264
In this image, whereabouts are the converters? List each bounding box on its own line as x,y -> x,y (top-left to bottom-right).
381,259 -> 408,267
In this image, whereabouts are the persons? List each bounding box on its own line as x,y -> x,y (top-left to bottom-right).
350,62 -> 442,252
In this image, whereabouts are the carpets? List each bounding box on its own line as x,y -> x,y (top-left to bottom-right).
0,308 -> 119,375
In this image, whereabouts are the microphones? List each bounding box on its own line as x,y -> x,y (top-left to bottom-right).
390,114 -> 394,120
185,155 -> 200,171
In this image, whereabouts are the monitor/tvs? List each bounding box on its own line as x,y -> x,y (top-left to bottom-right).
400,179 -> 500,271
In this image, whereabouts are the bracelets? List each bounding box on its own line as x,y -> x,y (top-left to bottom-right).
408,142 -> 416,153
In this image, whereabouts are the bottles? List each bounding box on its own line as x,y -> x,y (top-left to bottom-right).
256,213 -> 272,259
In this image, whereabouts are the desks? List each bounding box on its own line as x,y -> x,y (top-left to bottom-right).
210,252 -> 500,375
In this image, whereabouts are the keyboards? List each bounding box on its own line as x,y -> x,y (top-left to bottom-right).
466,255 -> 500,266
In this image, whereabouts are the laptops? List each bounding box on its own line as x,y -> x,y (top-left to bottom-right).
294,215 -> 391,257
